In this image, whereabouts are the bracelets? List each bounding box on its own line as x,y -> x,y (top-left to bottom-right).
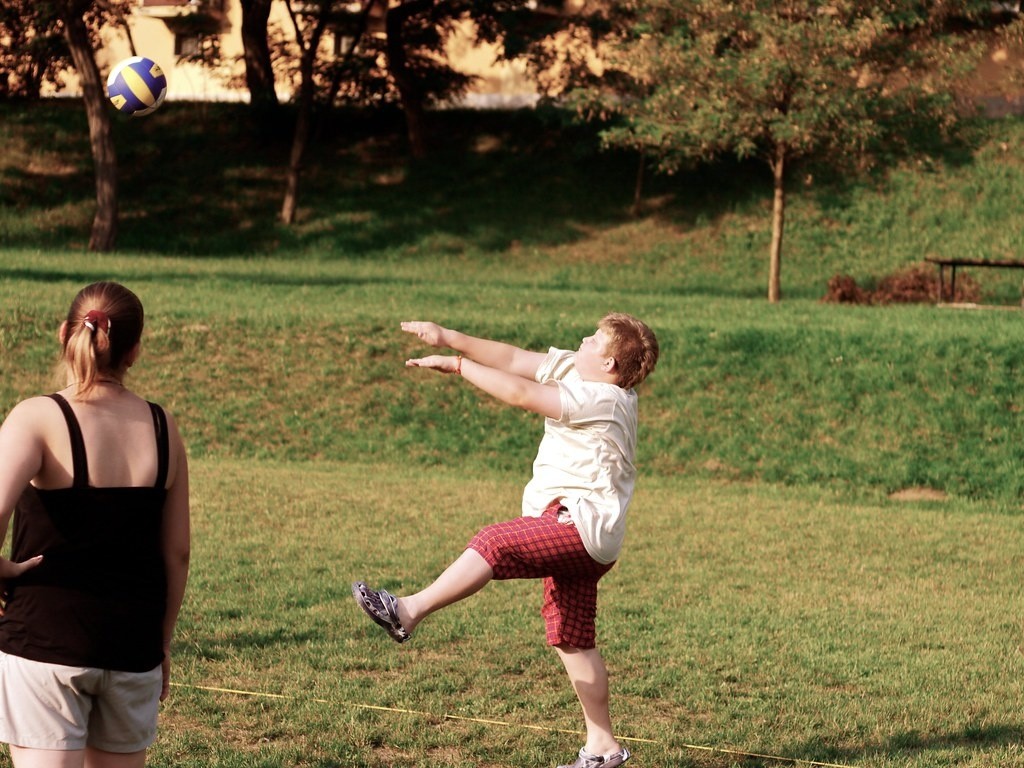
454,356 -> 462,375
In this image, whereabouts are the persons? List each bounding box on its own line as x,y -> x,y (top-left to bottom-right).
350,313 -> 661,767
0,282 -> 192,768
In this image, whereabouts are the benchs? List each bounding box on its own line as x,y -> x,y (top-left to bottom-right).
924,254 -> 1024,306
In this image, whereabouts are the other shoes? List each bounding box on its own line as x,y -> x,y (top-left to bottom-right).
556,747 -> 631,768
351,580 -> 412,643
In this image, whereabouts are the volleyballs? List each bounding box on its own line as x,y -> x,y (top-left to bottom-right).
106,56 -> 167,117
